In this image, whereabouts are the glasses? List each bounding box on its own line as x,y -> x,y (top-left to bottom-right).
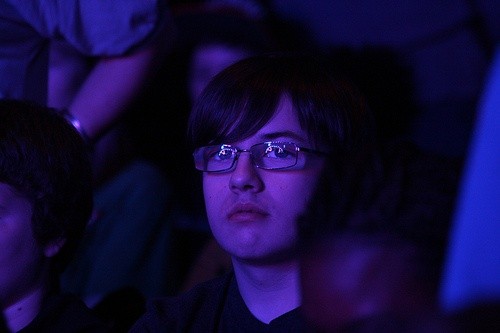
194,142 -> 324,171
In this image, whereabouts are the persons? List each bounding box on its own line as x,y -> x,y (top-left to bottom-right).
128,55 -> 460,332
1,0 -> 175,182
0,96 -> 146,333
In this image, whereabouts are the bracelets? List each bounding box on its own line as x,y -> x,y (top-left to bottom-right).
63,107 -> 90,143
